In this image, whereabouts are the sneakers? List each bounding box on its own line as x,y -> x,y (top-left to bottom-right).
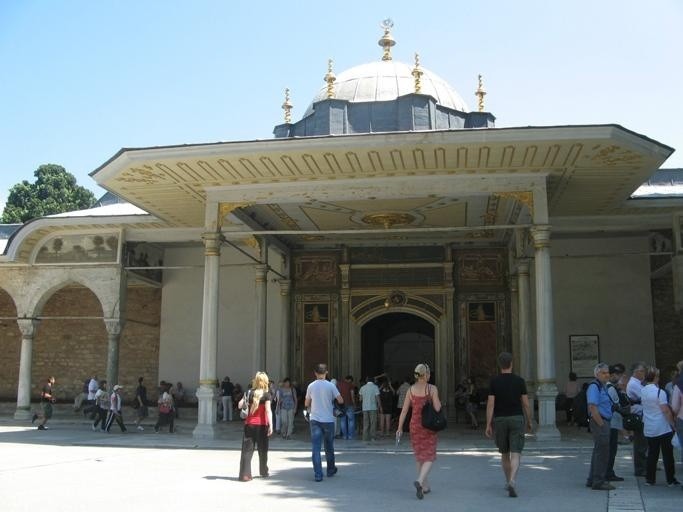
507,482 -> 518,497
38,425 -> 47,430
414,480 -> 423,499
32,414 -> 37,423
137,426 -> 144,430
586,474 -> 624,490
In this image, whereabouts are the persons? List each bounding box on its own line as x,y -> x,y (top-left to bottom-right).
583,357 -> 682,491
217,375 -> 298,441
31,374 -> 186,435
485,352 -> 533,498
237,372 -> 273,482
462,377 -> 483,429
304,362 -> 343,483
563,371 -> 583,426
326,374 -> 413,440
395,362 -> 441,499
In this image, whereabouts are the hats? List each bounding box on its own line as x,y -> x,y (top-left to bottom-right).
113,385 -> 123,390
632,363 -> 647,372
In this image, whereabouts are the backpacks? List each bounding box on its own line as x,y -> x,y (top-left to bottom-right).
569,382 -> 601,427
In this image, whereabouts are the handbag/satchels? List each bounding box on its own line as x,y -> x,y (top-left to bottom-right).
470,394 -> 480,403
240,402 -> 249,419
160,404 -> 172,413
422,400 -> 447,431
624,413 -> 642,429
619,389 -> 631,409
